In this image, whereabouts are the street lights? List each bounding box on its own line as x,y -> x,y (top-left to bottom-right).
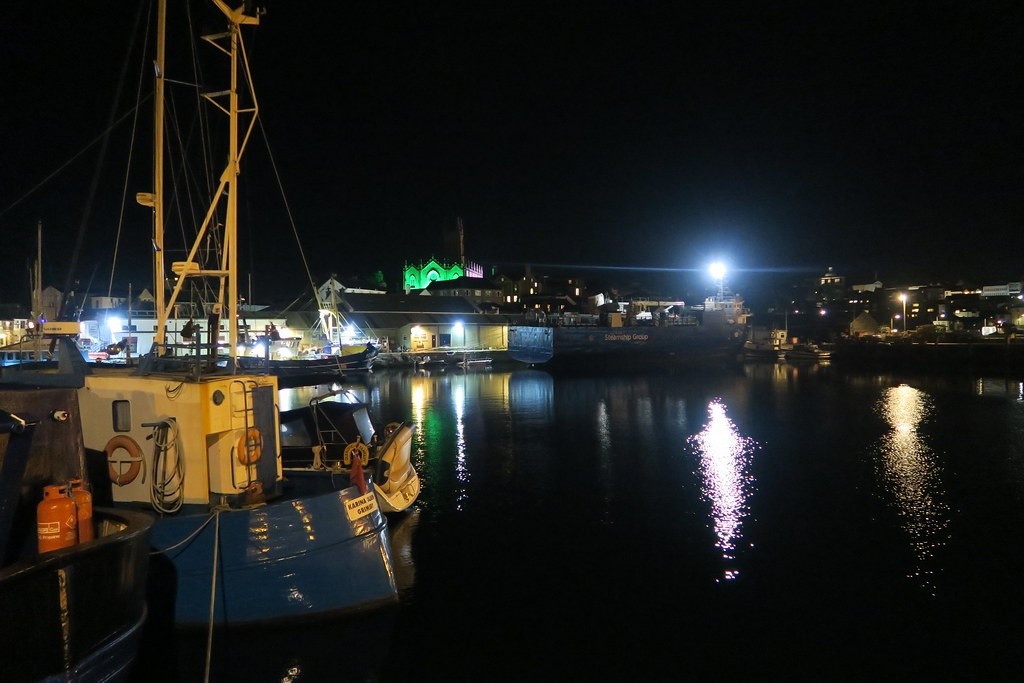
936,313 -> 945,346
899,294 -> 906,331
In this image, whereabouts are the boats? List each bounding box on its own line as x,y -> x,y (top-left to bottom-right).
508,298 -> 704,368
743,328 -> 793,354
0,0 -> 494,683
784,338 -> 831,361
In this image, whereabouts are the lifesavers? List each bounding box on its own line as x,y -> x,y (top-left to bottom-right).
238,429 -> 264,464
343,442 -> 369,466
104,435 -> 142,486
